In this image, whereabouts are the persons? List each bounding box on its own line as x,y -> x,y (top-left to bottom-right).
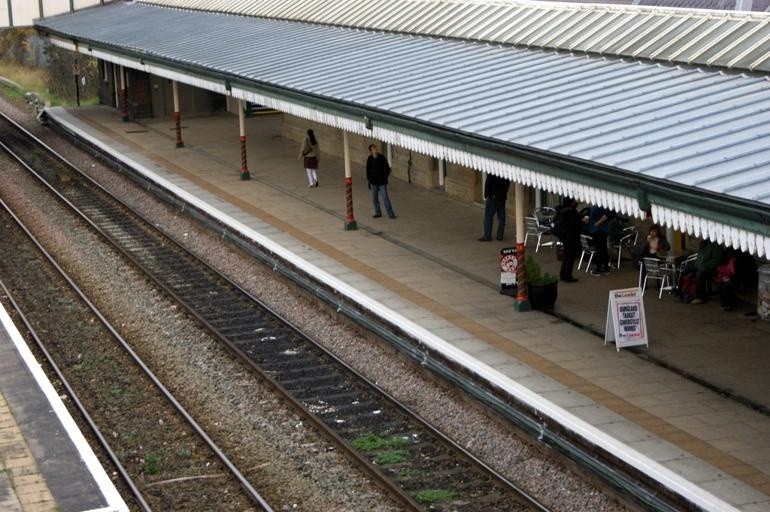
365,142 -> 397,219
297,128 -> 320,188
474,173 -> 510,241
547,195 -> 759,315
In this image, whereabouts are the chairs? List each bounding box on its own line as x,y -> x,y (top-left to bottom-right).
524,206 -> 697,300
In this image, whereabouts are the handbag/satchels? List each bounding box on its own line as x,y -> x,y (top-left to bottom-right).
301,138 -> 312,158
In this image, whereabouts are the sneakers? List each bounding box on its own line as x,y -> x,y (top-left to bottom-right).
476,233 -> 617,280
